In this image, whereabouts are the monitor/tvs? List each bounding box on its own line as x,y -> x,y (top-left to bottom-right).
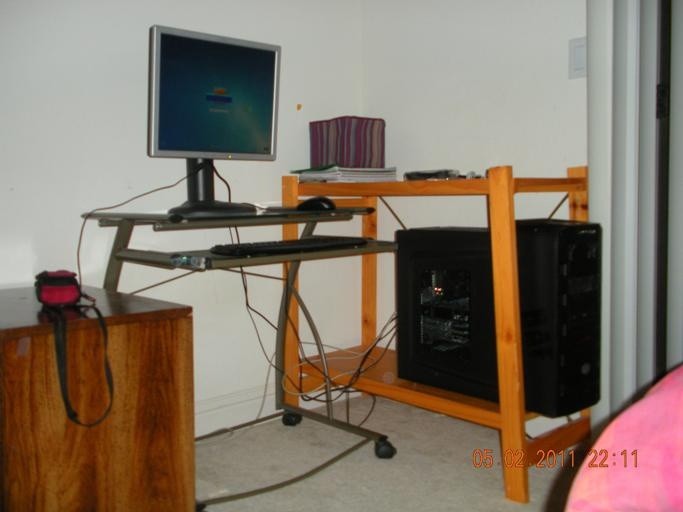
147,25 -> 282,222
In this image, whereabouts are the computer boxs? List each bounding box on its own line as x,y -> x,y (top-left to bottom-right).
396,220 -> 601,418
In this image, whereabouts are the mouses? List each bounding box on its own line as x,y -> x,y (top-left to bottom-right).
297,196 -> 336,211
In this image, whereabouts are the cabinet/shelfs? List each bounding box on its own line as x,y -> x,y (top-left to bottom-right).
281,167 -> 590,505
1,286 -> 196,512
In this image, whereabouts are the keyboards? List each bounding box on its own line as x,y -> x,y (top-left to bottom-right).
210,234 -> 368,257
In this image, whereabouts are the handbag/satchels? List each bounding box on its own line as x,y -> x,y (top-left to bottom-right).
35,270 -> 81,307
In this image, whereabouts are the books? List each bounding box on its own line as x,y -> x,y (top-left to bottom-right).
288,163 -> 399,185
